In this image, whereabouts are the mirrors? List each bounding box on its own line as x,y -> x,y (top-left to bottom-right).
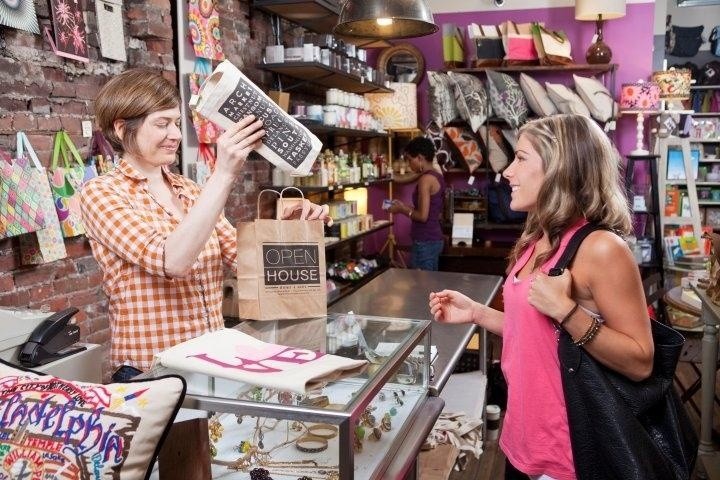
376,44 -> 427,89
650,1 -> 720,87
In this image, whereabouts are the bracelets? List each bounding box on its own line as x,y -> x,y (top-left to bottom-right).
573,317 -> 601,346
409,207 -> 413,218
559,303 -> 578,326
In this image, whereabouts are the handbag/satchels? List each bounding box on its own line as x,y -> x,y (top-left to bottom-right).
440,217 -> 453,235
664,11 -> 720,112
441,21 -> 572,66
47,130 -> 105,237
418,411 -> 486,461
186,0 -> 227,192
233,186 -> 330,320
44,0 -> 90,64
1,0 -> 40,37
1,130 -> 67,264
547,222 -> 703,479
93,0 -> 128,64
485,184 -> 530,224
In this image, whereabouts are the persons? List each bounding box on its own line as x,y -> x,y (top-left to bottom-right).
429,113 -> 655,480
79,70 -> 334,371
387,136 -> 446,271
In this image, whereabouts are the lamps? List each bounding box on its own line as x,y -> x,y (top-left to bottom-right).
618,77 -> 665,158
572,0 -> 628,65
331,0 -> 441,40
364,75 -> 419,272
652,64 -> 696,115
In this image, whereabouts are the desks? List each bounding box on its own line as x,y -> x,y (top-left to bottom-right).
662,282 -> 706,320
334,264 -> 507,480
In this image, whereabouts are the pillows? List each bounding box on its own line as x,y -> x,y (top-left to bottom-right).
0,356 -> 191,480
423,67 -> 623,185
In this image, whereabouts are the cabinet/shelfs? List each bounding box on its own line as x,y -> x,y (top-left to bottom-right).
654,85 -> 720,232
255,0 -> 396,306
130,305 -> 445,480
438,62 -> 618,233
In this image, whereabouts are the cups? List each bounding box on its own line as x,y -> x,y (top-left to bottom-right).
698,147 -> 720,203
260,36 -> 391,236
486,404 -> 501,441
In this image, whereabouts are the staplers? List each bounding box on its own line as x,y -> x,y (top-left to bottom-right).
19,307 -> 87,368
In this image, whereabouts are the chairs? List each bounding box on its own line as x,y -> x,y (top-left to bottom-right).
642,271 -> 719,420
